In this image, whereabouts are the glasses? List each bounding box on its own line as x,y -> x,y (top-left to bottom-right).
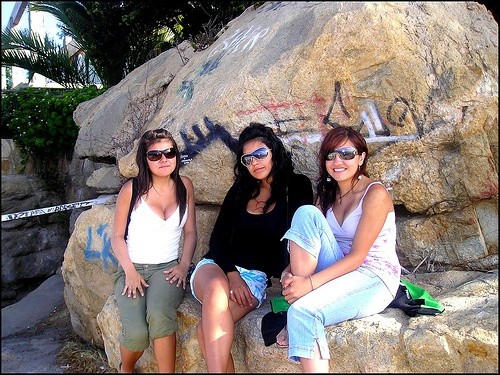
146,147 -> 176,161
325,146 -> 358,161
241,146 -> 271,167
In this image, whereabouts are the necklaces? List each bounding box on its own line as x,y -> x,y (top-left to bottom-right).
152,183 -> 174,195
339,175 -> 362,204
254,199 -> 266,209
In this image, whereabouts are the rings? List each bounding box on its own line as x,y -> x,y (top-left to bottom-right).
230,291 -> 232,294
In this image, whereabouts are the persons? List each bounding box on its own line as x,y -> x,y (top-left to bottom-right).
280,125 -> 401,373
111,128 -> 197,373
190,122 -> 313,373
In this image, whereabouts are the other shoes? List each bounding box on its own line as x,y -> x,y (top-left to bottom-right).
118,362 -> 122,373
276,325 -> 289,347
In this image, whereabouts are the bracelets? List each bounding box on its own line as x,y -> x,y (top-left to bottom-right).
310,275 -> 315,291
